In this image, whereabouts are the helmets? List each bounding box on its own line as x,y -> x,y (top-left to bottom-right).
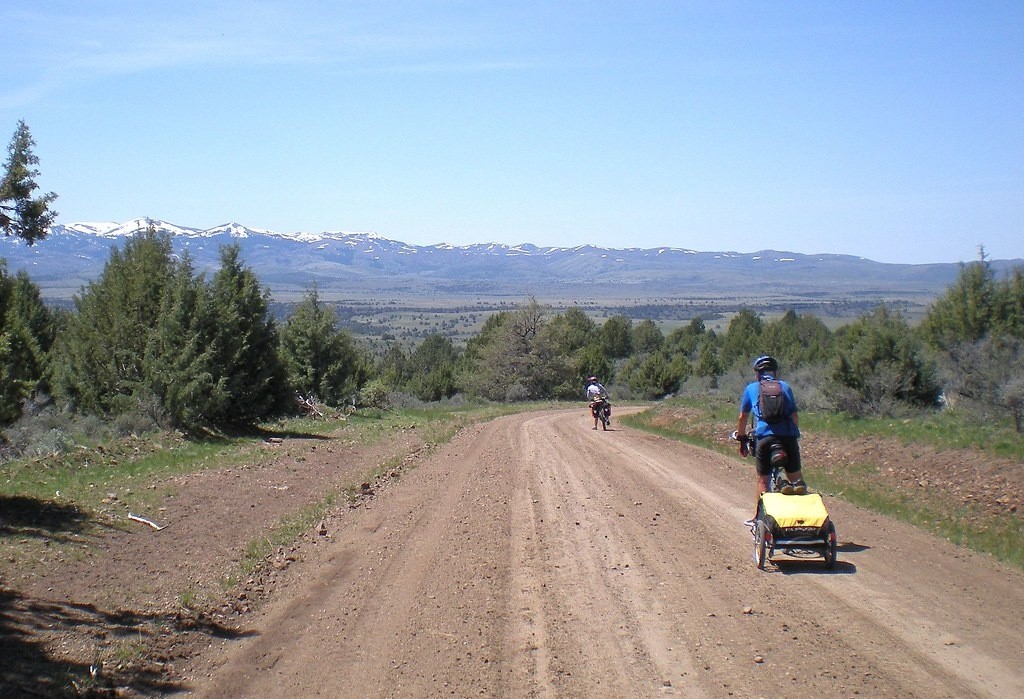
590,376 -> 597,381
753,355 -> 778,372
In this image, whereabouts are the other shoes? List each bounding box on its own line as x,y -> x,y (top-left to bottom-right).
592,427 -> 597,430
745,519 -> 755,526
607,420 -> 610,425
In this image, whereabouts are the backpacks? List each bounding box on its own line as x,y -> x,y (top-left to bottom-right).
757,377 -> 790,424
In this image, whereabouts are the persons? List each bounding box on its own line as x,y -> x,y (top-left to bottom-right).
736,355 -> 804,526
587,377 -> 610,430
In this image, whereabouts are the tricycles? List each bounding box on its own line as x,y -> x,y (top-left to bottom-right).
731,428 -> 837,570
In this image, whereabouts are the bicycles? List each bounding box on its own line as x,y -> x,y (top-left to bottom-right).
589,396 -> 611,431
